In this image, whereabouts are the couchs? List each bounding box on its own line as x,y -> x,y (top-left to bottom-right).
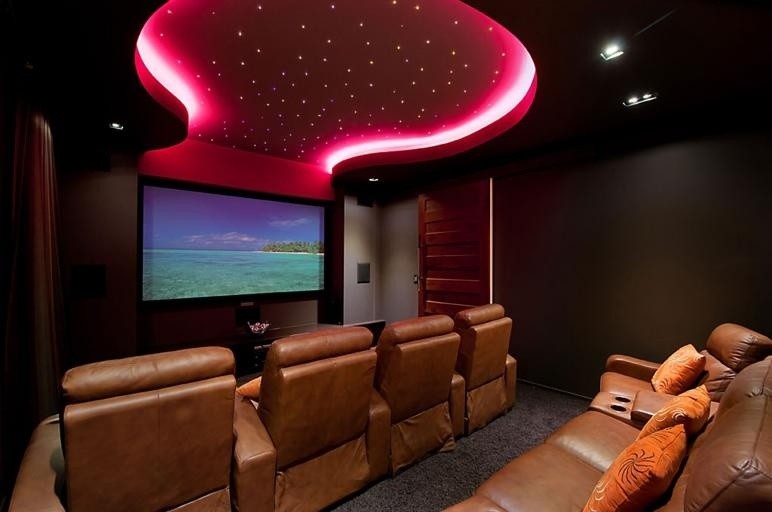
11,304 -> 517,512
446,322 -> 771,512
